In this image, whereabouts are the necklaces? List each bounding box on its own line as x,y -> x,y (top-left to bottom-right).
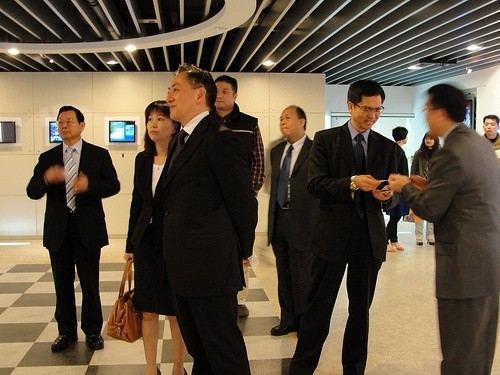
157,163 -> 161,170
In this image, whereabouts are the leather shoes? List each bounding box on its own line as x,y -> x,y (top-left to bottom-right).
51,332 -> 78,351
86,333 -> 104,349
271,320 -> 297,336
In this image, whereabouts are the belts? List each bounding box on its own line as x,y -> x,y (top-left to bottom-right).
275,202 -> 291,210
67,206 -> 78,214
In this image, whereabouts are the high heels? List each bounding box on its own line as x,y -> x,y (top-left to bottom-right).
390,242 -> 404,251
387,245 -> 395,253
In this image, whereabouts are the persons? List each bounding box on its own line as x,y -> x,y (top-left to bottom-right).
215,75 -> 266,317
124,100 -> 188,375
163,63 -> 258,375
287,80 -> 397,375
480,115 -> 500,164
271,106 -> 322,335
388,84 -> 500,375
26,105 -> 120,351
411,130 -> 441,244
384,126 -> 409,251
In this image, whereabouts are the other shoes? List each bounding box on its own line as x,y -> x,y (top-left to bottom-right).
238,303 -> 249,318
427,238 -> 436,245
183,368 -> 187,375
417,239 -> 424,246
156,368 -> 161,375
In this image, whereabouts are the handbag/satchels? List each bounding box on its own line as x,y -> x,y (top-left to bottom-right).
106,260 -> 142,342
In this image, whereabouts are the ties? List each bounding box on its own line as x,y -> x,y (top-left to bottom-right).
277,144 -> 293,207
166,128 -> 189,178
63,147 -> 76,211
354,134 -> 366,219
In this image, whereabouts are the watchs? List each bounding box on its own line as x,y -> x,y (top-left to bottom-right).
350,175 -> 359,191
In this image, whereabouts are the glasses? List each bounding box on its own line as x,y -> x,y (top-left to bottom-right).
352,100 -> 385,114
56,119 -> 79,126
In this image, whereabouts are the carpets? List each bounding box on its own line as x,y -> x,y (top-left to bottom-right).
0,262 -> 295,375
